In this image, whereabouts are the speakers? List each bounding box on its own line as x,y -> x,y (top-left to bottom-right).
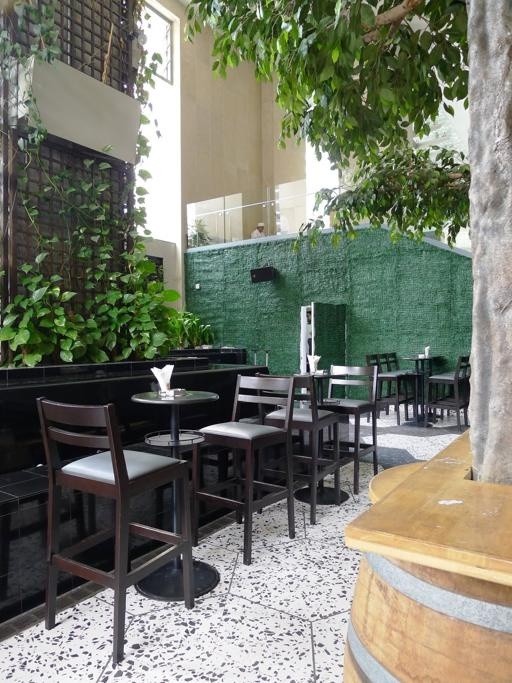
250,266 -> 274,283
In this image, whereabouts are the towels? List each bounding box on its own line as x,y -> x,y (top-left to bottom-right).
425,345 -> 430,357
305,354 -> 321,374
150,364 -> 175,392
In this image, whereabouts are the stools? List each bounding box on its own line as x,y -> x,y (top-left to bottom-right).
368,461 -> 428,501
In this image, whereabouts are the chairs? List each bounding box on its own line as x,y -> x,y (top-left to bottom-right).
192,374 -> 296,564
36,396 -> 188,662
308,366 -> 379,494
365,352 -> 470,432
255,372 -> 340,525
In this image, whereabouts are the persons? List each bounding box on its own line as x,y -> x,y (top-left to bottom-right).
251,224 -> 266,238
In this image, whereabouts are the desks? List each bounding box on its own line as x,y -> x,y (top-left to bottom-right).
292,371 -> 348,505
131,391 -> 219,601
